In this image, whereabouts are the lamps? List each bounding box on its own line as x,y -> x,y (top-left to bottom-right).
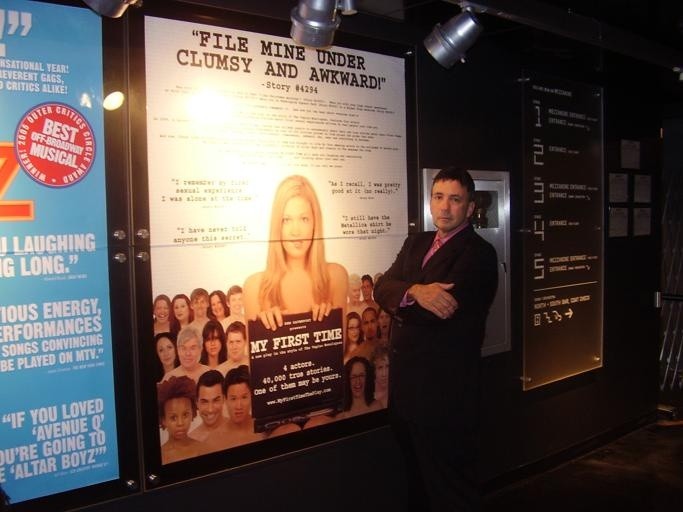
85,1 -> 487,72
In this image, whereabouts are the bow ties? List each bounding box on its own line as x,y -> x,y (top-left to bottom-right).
432,240 -> 443,253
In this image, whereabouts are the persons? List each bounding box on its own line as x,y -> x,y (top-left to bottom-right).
242,174 -> 348,439
152,286 -> 258,463
373,167 -> 499,511
332,272 -> 388,420
